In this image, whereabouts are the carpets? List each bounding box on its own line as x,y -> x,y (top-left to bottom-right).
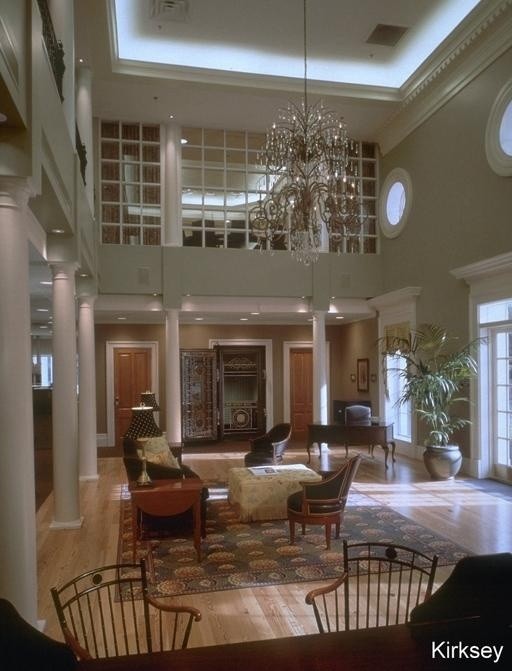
113,481 -> 477,602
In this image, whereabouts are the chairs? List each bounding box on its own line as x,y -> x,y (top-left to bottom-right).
50,556 -> 202,661
123,457 -> 209,538
306,540 -> 439,637
286,453 -> 362,550
344,405 -> 373,457
245,421 -> 293,465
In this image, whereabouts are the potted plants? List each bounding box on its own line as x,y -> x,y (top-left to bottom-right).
372,321 -> 487,481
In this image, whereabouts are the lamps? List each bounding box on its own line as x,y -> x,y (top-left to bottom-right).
250,0 -> 368,266
125,407 -> 161,487
140,392 -> 160,411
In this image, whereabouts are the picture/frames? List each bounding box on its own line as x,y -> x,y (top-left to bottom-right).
356,358 -> 370,391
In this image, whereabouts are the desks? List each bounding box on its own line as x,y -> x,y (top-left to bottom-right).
307,422 -> 397,469
232,463 -> 322,523
78,625 -> 452,671
